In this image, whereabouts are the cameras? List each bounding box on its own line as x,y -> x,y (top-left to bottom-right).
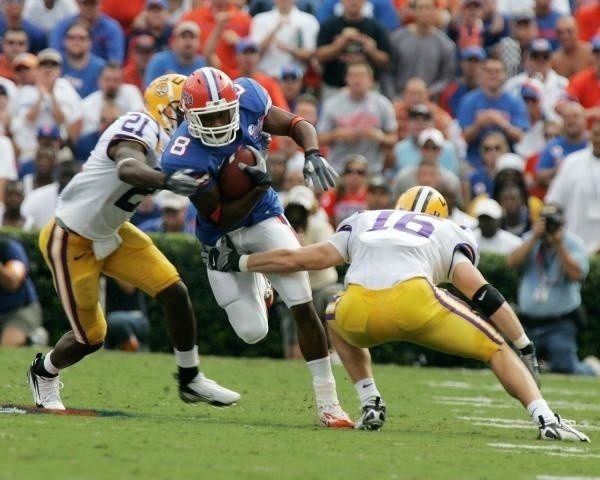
544,215 -> 560,233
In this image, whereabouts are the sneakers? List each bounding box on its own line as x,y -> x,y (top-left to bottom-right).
27,352 -> 66,412
283,340 -> 306,361
318,407 -> 356,430
354,396 -> 386,431
536,413 -> 590,445
178,374 -> 240,407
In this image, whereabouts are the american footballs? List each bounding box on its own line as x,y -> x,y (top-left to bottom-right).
223,149 -> 256,198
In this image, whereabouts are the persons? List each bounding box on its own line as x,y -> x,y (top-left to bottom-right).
157,65 -> 359,431
199,183 -> 595,442
0,2 -> 599,380
27,72 -> 241,410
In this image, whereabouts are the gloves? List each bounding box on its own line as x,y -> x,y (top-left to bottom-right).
263,281 -> 275,311
200,235 -> 242,273
518,343 -> 542,391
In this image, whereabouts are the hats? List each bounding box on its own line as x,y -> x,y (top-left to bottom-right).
0,1 -> 599,220
